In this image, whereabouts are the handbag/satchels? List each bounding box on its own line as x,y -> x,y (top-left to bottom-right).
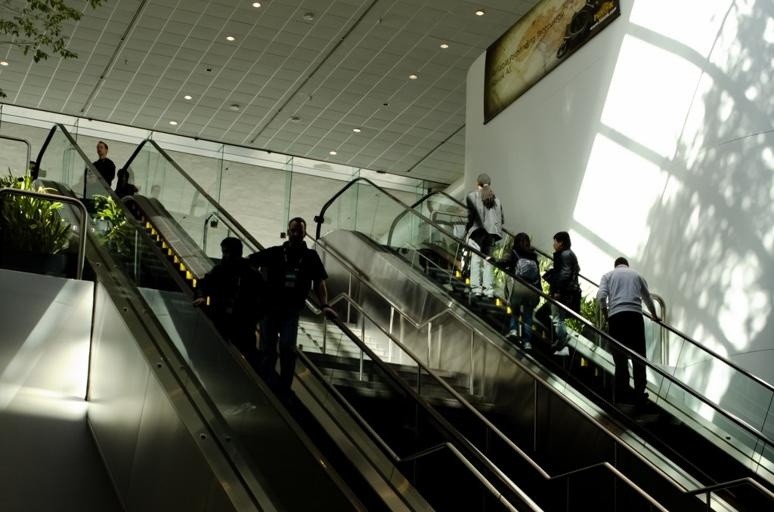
459,253 -> 470,275
560,280 -> 580,318
467,228 -> 490,255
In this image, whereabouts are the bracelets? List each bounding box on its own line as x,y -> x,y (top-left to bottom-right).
320,303 -> 330,309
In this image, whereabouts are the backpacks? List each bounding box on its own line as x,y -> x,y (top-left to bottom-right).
513,257 -> 539,297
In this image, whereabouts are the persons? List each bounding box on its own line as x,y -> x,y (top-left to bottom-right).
464,172 -> 506,298
151,184 -> 162,198
535,231 -> 579,355
244,217 -> 338,394
117,168 -> 141,195
192,235 -> 266,376
91,141 -> 115,197
485,231 -> 542,349
596,256 -> 663,403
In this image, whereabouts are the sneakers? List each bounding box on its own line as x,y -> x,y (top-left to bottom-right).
554,346 -> 570,356
505,329 -> 532,350
470,288 -> 495,299
